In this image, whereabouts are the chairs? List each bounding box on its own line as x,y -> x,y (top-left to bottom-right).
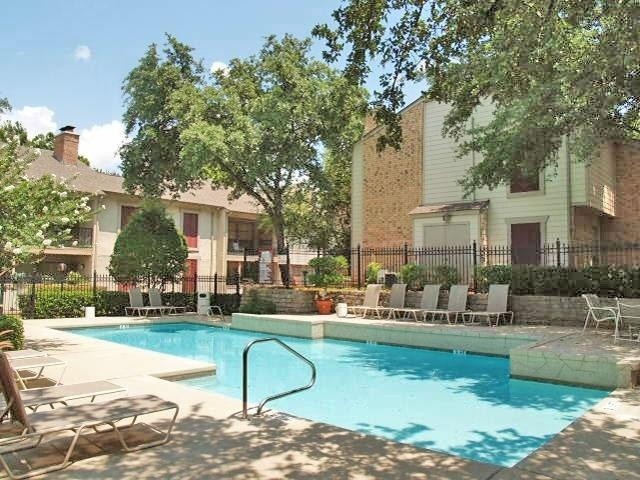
581,294 -> 640,346
348,283 -> 514,328
0,344 -> 180,480
124,288 -> 186,318
197,291 -> 224,322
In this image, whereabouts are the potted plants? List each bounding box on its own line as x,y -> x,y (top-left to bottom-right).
306,255 -> 350,314
335,291 -> 348,317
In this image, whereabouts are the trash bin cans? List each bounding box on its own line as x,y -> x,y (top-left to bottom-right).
198,292 -> 210,316
